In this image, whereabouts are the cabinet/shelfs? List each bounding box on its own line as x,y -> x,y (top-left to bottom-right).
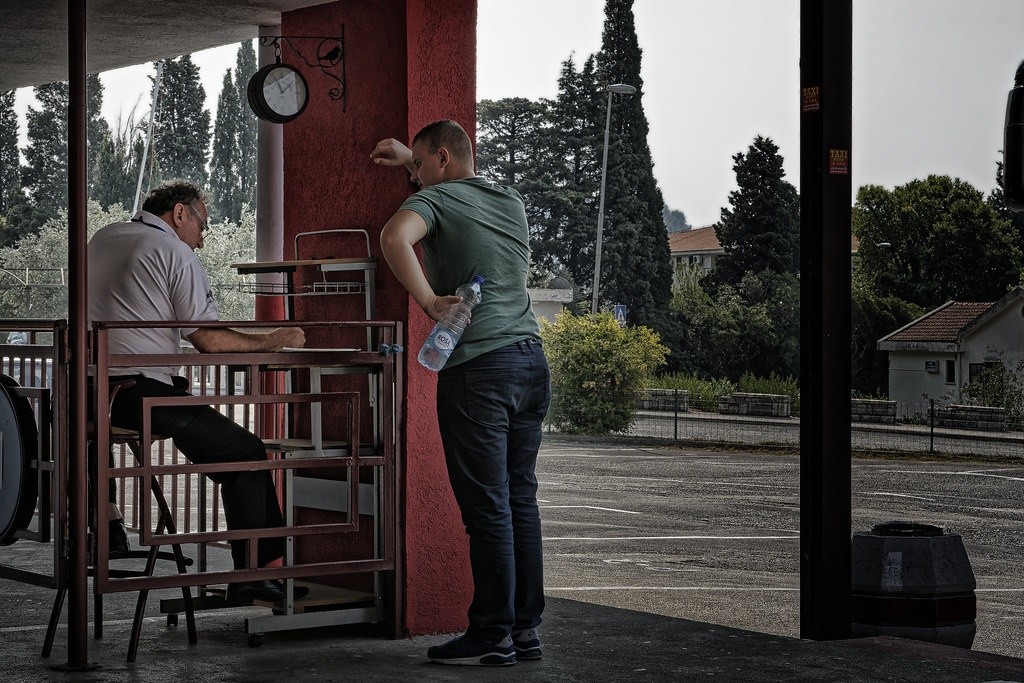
158,228 -> 392,647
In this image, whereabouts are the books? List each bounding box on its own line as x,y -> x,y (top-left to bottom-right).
275,347 -> 362,353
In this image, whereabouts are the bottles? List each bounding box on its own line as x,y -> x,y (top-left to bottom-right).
417,275 -> 484,371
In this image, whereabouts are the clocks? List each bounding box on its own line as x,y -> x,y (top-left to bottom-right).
246,22 -> 347,125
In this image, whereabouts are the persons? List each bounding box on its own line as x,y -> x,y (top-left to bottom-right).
6,331 -> 28,345
368,118 -> 553,668
49,182 -> 308,603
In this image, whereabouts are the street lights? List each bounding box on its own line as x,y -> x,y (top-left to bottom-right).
592,82 -> 638,314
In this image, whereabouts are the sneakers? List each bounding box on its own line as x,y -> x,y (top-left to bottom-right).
427,631 -> 519,667
512,626 -> 542,660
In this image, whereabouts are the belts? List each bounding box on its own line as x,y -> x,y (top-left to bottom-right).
509,339 -> 542,346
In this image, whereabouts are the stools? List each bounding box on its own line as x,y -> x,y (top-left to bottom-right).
39,428 -> 199,663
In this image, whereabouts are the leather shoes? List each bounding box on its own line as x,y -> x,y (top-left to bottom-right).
107,522 -> 130,552
226,579 -> 310,604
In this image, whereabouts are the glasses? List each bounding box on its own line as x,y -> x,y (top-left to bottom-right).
185,201 -> 213,239
